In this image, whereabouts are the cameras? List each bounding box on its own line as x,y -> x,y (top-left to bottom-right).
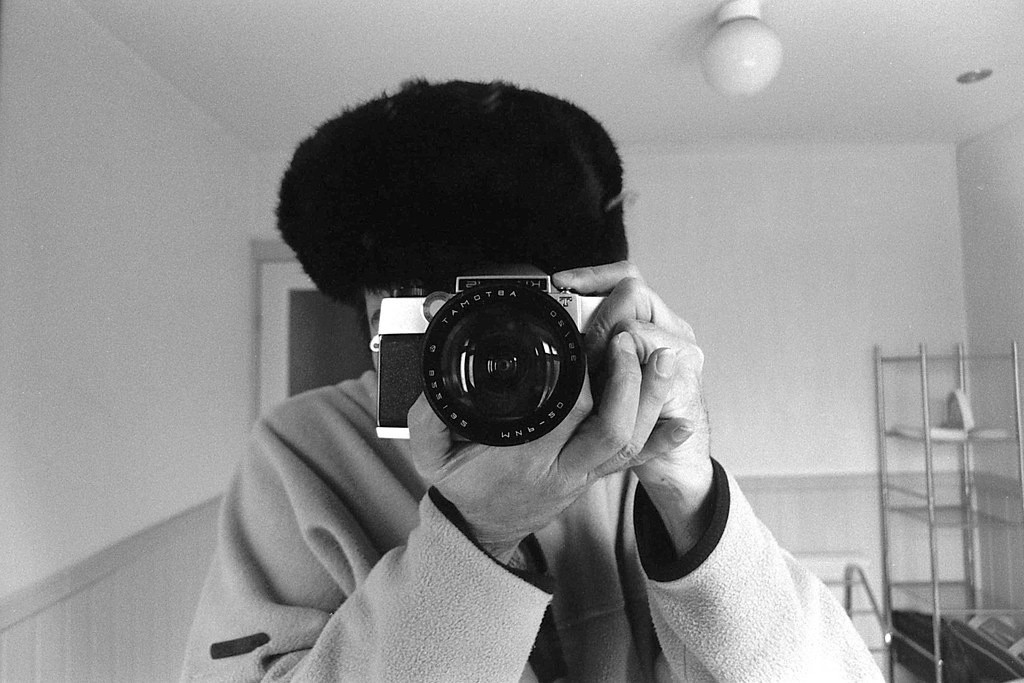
369,262 -> 609,446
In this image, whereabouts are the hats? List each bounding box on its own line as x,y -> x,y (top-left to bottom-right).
275,81 -> 629,295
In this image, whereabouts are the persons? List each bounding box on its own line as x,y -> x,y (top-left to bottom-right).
181,76 -> 886,683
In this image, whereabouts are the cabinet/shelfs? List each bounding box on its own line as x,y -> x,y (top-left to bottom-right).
872,341 -> 1024,683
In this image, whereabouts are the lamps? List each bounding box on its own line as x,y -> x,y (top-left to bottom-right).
706,0 -> 782,97
